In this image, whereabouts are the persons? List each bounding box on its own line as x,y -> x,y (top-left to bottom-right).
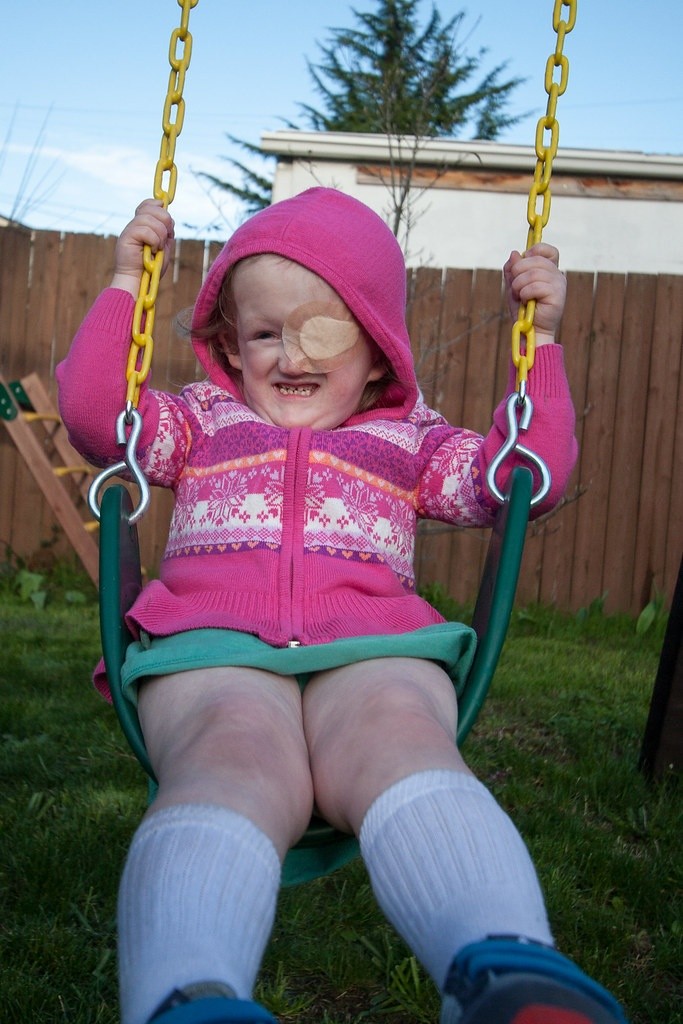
55,186 -> 628,1023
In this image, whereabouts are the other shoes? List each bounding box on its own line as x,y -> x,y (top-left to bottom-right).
143,982 -> 278,1024
436,934 -> 626,1024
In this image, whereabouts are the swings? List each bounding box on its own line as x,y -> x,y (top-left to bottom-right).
91,0 -> 578,847
